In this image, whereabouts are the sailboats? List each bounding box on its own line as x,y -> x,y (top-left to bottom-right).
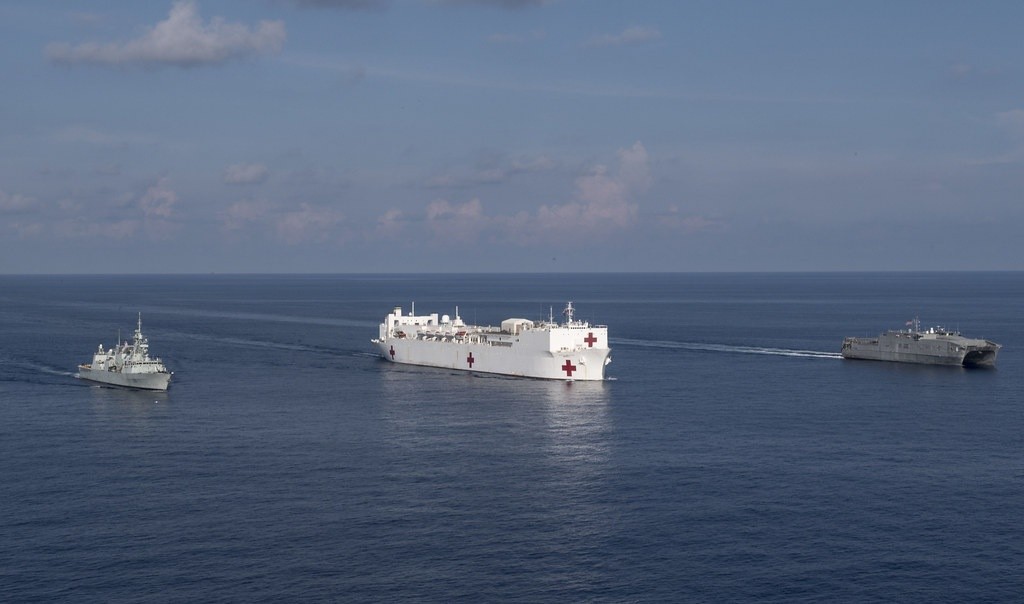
78,311 -> 173,390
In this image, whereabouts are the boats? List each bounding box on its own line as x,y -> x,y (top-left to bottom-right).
370,295 -> 611,380
840,315 -> 1001,366
